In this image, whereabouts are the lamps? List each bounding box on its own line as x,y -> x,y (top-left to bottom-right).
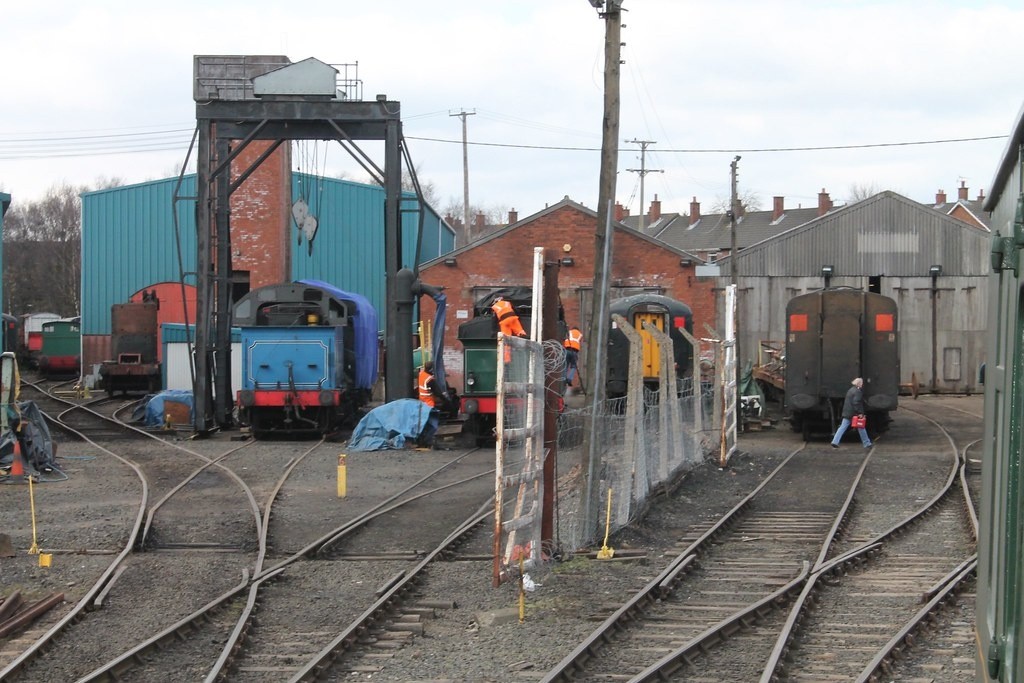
445,257 -> 456,266
822,265 -> 833,276
680,259 -> 692,266
930,264 -> 942,275
562,258 -> 574,266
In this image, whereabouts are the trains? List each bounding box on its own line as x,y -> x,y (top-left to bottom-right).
15,308 -> 81,381
230,281 -> 379,440
456,308 -> 571,449
582,291 -> 695,415
781,284 -> 902,442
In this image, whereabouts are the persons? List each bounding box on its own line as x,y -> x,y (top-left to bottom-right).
417,360 -> 445,449
831,378 -> 873,448
563,325 -> 584,387
491,296 -> 526,365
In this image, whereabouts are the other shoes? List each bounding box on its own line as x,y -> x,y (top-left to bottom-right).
566,379 -> 573,387
831,443 -> 840,448
